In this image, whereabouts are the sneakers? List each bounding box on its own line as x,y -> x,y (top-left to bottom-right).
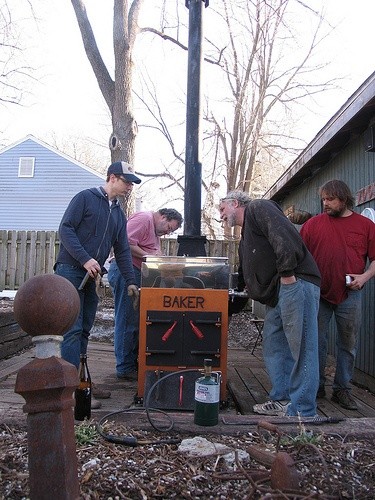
316,387 -> 326,398
253,400 -> 291,416
330,390 -> 359,410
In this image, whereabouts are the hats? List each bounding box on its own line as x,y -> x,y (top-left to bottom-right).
107,161 -> 141,184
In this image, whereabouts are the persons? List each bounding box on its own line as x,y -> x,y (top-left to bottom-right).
53,161 -> 139,408
107,208 -> 184,380
299,180 -> 375,411
219,191 -> 322,417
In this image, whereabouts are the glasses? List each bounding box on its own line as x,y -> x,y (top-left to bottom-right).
116,175 -> 134,186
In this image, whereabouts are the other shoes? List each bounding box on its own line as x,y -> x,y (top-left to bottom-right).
117,372 -> 138,380
90,382 -> 111,397
72,392 -> 101,409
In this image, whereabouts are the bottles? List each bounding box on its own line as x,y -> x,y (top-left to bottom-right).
74,353 -> 93,421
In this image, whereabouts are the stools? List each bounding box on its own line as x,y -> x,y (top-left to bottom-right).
249,319 -> 264,355
